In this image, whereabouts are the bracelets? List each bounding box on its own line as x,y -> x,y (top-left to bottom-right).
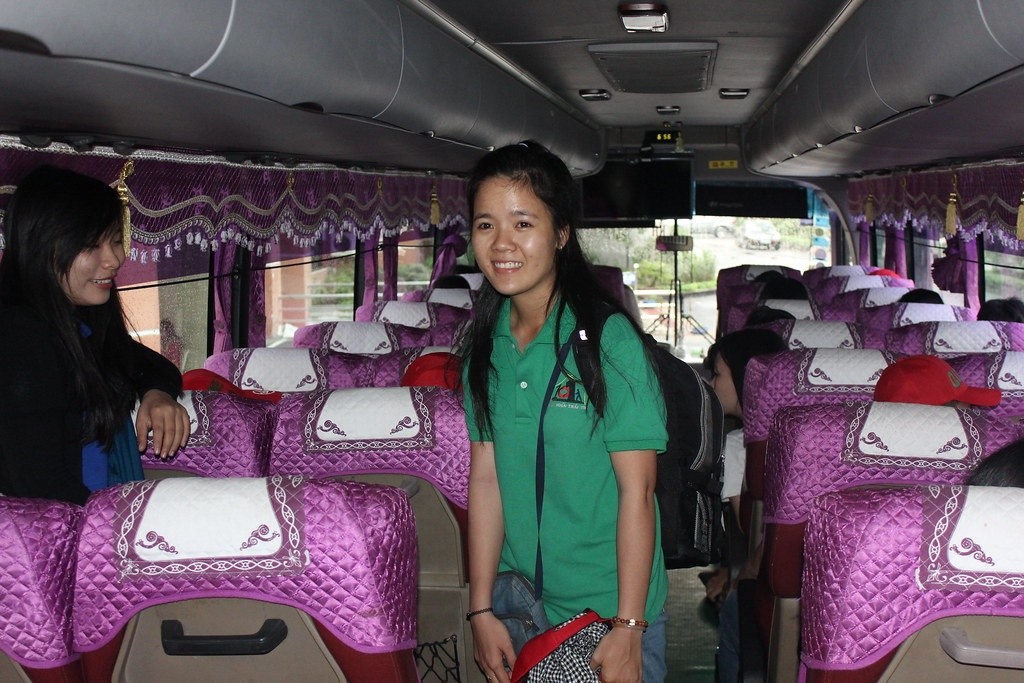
464,606 -> 494,620
609,616 -> 650,633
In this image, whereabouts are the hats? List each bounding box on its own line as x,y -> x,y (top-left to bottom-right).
510,609 -> 615,683
869,269 -> 900,278
182,369 -> 281,401
401,352 -> 467,389
874,354 -> 1000,407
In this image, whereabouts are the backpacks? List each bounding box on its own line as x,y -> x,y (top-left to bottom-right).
471,292 -> 726,570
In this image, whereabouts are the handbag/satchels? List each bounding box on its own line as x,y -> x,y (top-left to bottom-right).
482,570 -> 550,670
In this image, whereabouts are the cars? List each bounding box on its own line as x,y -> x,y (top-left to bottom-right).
661,215 -> 735,237
736,220 -> 782,249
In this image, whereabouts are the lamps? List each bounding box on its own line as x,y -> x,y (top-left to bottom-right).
618,3 -> 672,35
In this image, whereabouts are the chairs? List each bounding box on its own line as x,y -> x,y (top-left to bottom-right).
118,390 -> 275,481
0,496 -> 86,683
203,265 -> 642,445
794,483 -> 1024,683
716,264 -> 1024,461
767,402 -> 1020,683
267,387 -> 491,683
72,474 -> 422,683
735,347 -> 912,683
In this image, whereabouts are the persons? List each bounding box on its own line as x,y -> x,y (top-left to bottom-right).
895,287 -> 945,304
741,303 -> 795,326
430,275 -> 470,288
759,277 -> 809,300
441,139 -> 670,683
974,295 -> 1023,322
0,167 -> 191,506
747,269 -> 785,283
701,320 -> 801,607
158,319 -> 183,369
